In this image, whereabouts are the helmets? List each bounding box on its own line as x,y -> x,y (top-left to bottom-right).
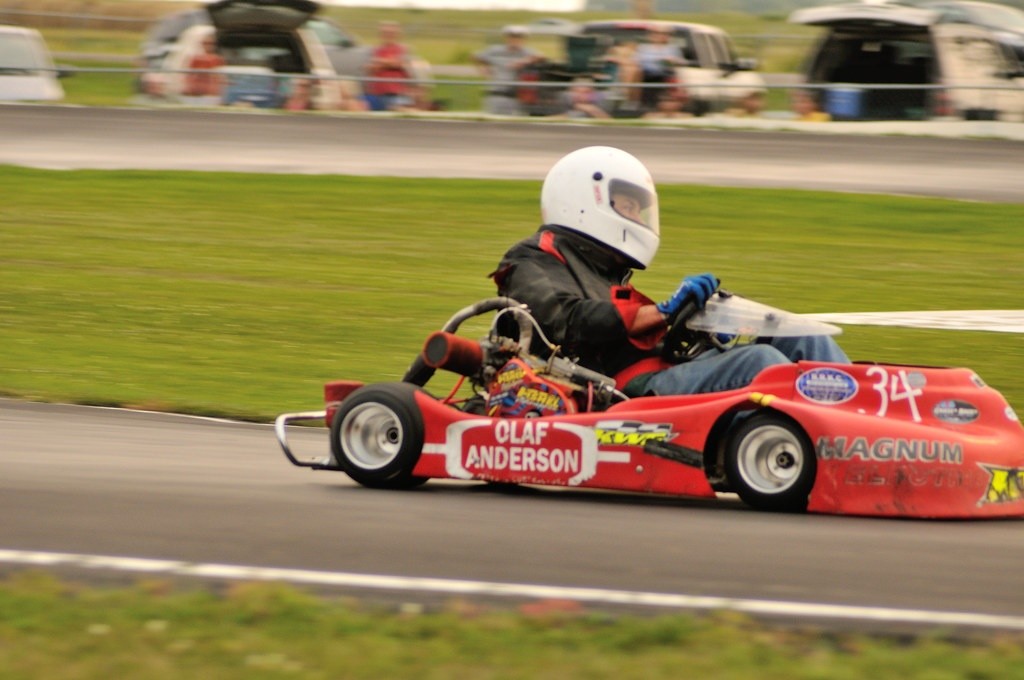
540,146 -> 661,270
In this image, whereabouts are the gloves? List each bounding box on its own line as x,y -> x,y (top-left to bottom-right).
656,272 -> 718,321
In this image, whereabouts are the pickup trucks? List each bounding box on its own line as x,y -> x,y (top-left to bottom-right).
516,18 -> 768,118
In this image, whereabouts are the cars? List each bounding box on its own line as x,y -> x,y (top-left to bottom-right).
788,0 -> 1024,123
0,0 -> 436,109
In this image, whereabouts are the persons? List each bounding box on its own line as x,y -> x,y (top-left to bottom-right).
637,92 -> 695,120
702,70 -> 769,119
786,88 -> 831,123
338,20 -> 436,111
561,22 -> 688,122
138,20 -> 341,111
488,146 -> 854,399
474,24 -> 549,116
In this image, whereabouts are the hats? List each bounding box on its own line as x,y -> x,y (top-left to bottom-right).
501,24 -> 529,36
